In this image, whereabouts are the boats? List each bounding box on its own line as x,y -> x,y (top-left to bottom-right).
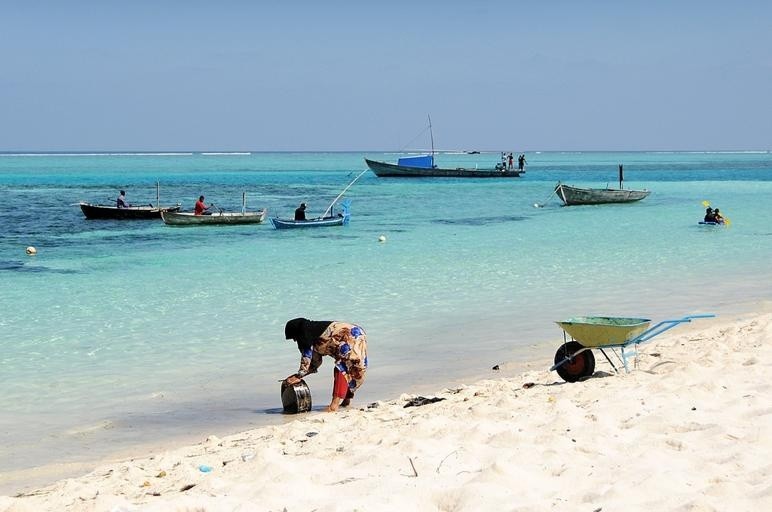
363,114 -> 526,177
698,220 -> 727,225
270,212 -> 345,228
79,182 -> 266,224
554,165 -> 651,205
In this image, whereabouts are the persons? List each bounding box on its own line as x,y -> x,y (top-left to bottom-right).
704,206 -> 722,223
117,190 -> 129,209
195,195 -> 216,217
283,317 -> 368,414
294,203 -> 308,223
495,151 -> 527,171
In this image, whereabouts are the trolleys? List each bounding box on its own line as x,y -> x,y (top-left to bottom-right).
550,314 -> 717,383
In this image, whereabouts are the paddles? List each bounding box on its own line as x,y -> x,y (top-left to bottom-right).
700,201 -> 730,225
